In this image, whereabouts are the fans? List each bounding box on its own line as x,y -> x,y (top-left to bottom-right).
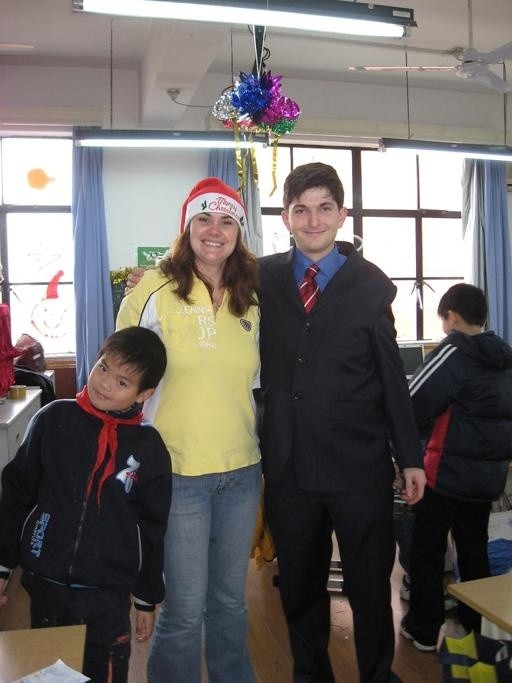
348,1 -> 512,95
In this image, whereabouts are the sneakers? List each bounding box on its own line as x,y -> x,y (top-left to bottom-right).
398,613 -> 439,653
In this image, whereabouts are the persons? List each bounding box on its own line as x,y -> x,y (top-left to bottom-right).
400,283 -> 512,653
124,161 -> 429,683
113,177 -> 274,682
1,326 -> 171,682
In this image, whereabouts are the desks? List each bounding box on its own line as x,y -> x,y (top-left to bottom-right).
0,624 -> 86,683
0,368 -> 56,493
448,571 -> 512,640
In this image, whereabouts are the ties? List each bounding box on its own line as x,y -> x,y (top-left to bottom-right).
297,263 -> 325,318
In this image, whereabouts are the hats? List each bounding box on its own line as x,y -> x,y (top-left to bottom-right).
178,175 -> 250,236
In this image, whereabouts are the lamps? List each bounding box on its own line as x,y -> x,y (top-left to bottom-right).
71,0 -> 419,38
379,32 -> 512,163
72,22 -> 269,150
